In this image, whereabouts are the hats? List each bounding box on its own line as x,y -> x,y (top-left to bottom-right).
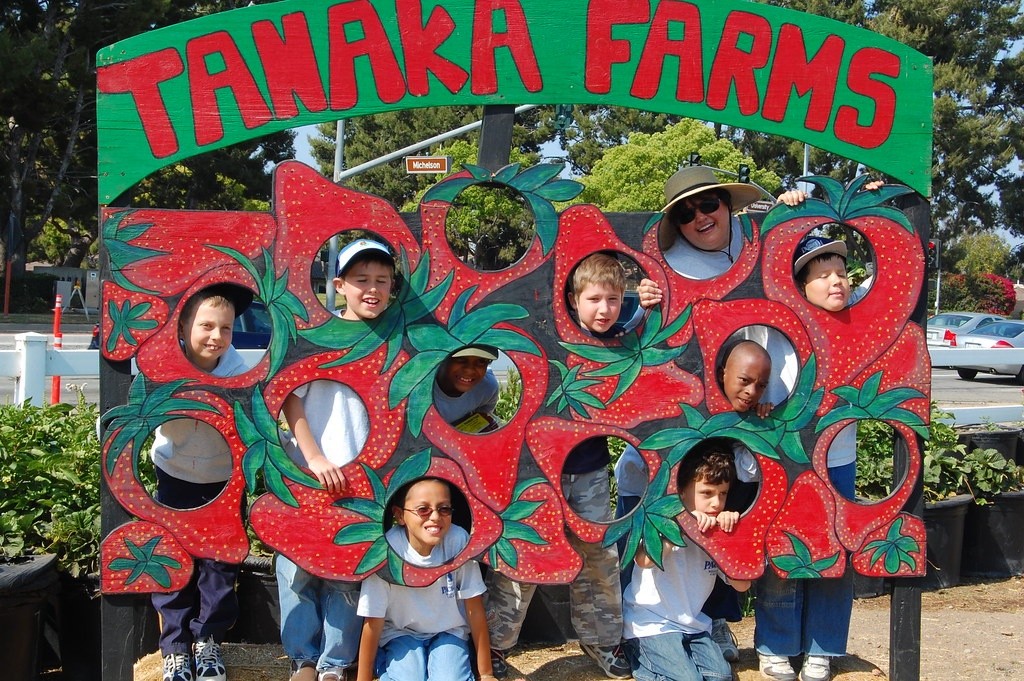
660,166 -> 761,212
335,239 -> 394,278
792,236 -> 847,276
452,345 -> 499,360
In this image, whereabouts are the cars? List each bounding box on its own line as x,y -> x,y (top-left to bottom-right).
926,313 -> 1006,350
950,321 -> 1024,384
89,301 -> 272,350
611,290 -> 640,331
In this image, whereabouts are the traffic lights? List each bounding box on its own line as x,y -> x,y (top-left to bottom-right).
928,239 -> 940,269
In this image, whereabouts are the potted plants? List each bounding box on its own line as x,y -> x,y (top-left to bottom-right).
0,397 -> 1023,681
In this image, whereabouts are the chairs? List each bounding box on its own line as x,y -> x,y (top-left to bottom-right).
935,318 -> 946,325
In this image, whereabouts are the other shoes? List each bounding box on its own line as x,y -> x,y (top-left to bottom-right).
289,659 -> 347,681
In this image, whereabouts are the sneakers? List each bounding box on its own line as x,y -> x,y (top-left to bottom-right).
490,648 -> 508,680
756,650 -> 796,681
711,618 -> 739,662
580,643 -> 631,679
164,634 -> 227,681
798,654 -> 830,681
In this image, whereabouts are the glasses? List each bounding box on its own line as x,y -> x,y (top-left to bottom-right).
673,197 -> 720,224
402,505 -> 454,518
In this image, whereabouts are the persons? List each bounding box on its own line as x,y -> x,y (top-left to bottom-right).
356,480 -> 498,681
381,345 -> 498,540
623,447 -> 741,681
149,291 -> 251,681
743,235 -> 871,681
482,253 -> 662,681
615,340 -> 772,663
274,239 -> 398,681
658,166 -> 805,406
